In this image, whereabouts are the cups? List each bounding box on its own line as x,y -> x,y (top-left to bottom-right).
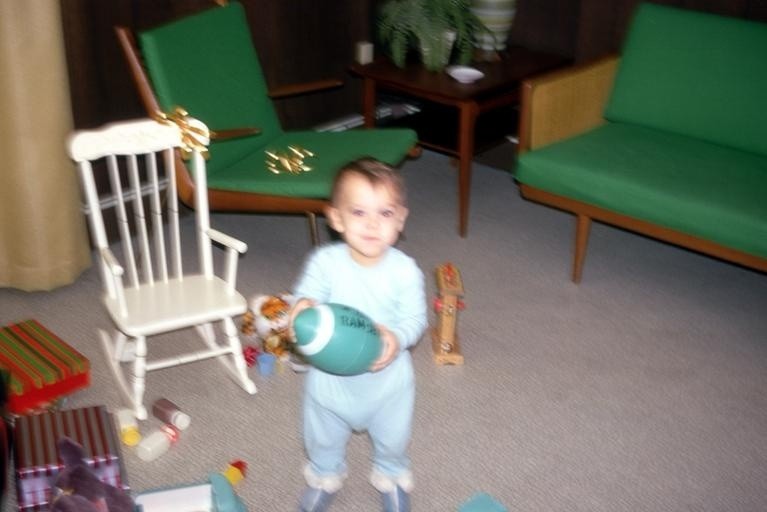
257,353 -> 275,376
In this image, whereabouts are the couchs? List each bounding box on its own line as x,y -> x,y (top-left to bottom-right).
509,0 -> 767,284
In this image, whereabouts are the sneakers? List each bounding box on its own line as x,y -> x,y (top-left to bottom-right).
385,486 -> 409,512
299,487 -> 331,512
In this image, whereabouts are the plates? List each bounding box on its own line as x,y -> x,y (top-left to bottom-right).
447,68 -> 483,85
454,492 -> 505,511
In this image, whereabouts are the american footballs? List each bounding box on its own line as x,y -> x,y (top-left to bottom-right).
293,303 -> 383,377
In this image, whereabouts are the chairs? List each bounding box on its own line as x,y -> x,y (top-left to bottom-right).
65,115 -> 259,422
112,1 -> 423,248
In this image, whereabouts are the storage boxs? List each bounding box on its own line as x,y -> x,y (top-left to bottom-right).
0,317 -> 91,415
11,403 -> 131,512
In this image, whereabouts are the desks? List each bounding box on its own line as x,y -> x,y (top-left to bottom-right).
349,47 -> 576,240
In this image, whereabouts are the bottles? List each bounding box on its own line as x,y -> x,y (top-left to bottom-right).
116,399 -> 191,464
226,461 -> 246,486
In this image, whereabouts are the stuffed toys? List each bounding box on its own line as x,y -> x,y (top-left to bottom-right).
241,289 -> 307,374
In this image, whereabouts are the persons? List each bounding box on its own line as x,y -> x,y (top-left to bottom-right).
287,156 -> 428,512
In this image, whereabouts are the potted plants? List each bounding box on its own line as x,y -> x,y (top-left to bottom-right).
375,1 -> 500,74
